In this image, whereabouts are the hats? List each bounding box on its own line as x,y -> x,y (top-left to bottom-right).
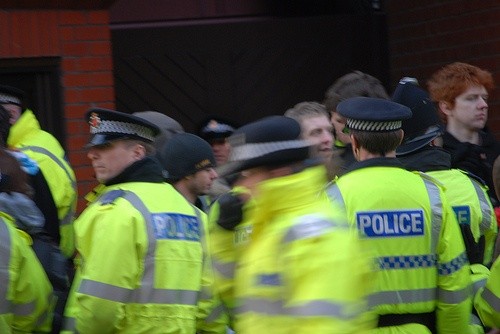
392,77 -> 441,155
0,84 -> 28,106
220,115 -> 309,176
196,116 -> 238,139
154,132 -> 216,183
336,97 -> 413,132
82,108 -> 161,148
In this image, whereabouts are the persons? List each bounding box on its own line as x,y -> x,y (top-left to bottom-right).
322,97 -> 471,334
1,62 -> 500,334
211,116 -> 367,333
60,109 -> 218,334
